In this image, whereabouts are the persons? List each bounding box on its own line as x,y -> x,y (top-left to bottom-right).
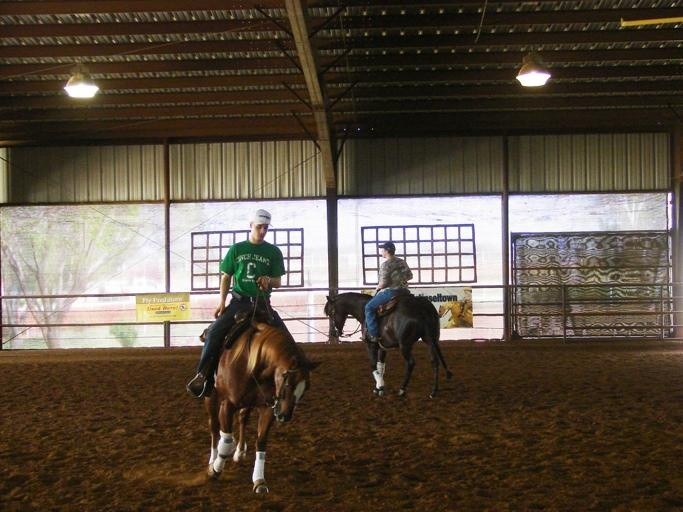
359,241 -> 418,344
187,209 -> 305,400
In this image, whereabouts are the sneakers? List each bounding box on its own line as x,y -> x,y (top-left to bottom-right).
191,378 -> 214,393
361,336 -> 377,343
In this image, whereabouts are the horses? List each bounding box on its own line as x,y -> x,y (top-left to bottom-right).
204,318 -> 325,495
438,301 -> 473,328
324,292 -> 454,400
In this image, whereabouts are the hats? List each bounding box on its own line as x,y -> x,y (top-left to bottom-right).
251,210 -> 272,226
378,242 -> 395,253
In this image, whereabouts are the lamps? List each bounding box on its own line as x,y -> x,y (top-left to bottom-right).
516,53 -> 551,87
64,63 -> 99,99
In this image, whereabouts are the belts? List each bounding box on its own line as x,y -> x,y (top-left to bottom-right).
243,296 -> 270,304
